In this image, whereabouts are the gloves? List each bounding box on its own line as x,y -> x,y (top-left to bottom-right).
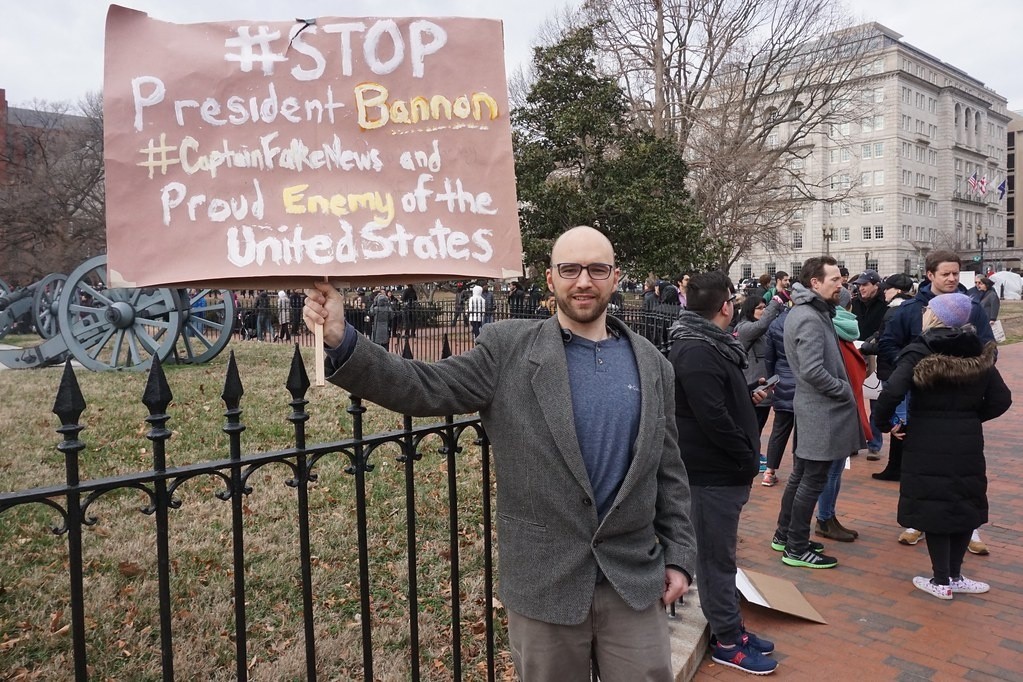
865,331 -> 880,344
861,342 -> 880,356
773,290 -> 791,306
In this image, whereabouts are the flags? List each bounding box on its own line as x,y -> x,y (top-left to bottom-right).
967,171 -> 1005,200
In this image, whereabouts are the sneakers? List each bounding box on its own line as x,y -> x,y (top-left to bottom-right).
712,629 -> 779,676
898,527 -> 926,545
771,528 -> 824,553
968,529 -> 990,555
781,541 -> 839,568
710,619 -> 774,655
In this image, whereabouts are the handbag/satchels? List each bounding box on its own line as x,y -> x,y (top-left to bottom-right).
863,371 -> 884,399
990,320 -> 1006,344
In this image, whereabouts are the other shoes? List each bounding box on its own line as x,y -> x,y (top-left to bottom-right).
815,516 -> 858,542
758,456 -> 768,472
912,574 -> 953,600
949,574 -> 990,594
761,472 -> 779,486
866,449 -> 900,482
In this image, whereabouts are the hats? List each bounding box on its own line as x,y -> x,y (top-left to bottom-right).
852,269 -> 882,285
982,278 -> 995,290
928,292 -> 973,329
837,287 -> 851,308
879,273 -> 913,292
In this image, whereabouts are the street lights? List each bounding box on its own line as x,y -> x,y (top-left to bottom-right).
822,223 -> 834,256
976,226 -> 989,275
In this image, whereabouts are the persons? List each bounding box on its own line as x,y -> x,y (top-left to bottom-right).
669,269 -> 779,675
872,293 -> 1014,599
770,256 -> 869,569
303,224 -> 699,682
8,270 -> 999,555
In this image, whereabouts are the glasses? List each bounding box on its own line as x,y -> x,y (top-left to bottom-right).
717,294 -> 737,313
552,262 -> 615,280
755,305 -> 767,311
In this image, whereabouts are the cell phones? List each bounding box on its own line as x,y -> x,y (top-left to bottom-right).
752,374 -> 780,394
